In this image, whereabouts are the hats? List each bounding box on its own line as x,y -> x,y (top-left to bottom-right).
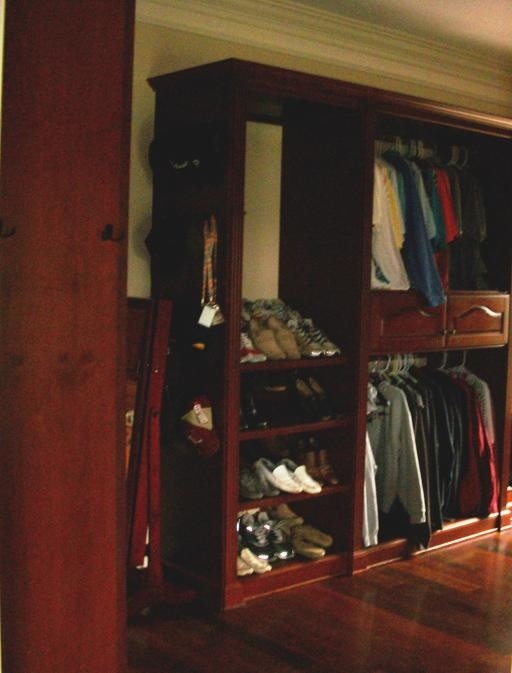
180,394 -> 221,464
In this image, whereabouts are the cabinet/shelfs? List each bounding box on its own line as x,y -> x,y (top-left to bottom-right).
144,56 -> 511,613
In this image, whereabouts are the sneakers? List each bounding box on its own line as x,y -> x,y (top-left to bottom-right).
239,376 -> 338,431
239,298 -> 341,364
240,436 -> 339,500
237,504 -> 333,577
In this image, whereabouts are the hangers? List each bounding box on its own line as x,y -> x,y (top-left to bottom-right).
377,133 -> 478,177
372,348 -> 485,401
